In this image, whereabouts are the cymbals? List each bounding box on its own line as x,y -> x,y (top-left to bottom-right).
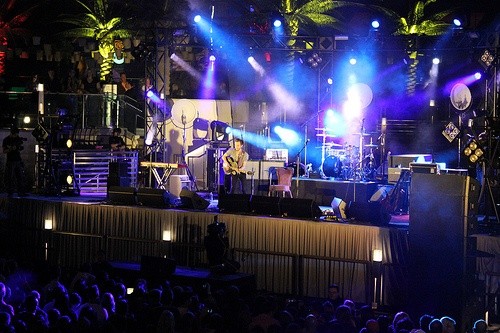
322,142 -> 341,147
364,144 -> 379,147
352,132 -> 371,137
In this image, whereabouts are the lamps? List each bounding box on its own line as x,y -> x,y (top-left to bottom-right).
303,50 -> 328,72
478,47 -> 498,70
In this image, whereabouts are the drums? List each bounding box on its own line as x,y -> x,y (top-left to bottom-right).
328,149 -> 346,159
346,144 -> 359,160
321,155 -> 342,177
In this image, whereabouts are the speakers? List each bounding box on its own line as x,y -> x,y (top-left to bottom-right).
136,188 -> 177,209
108,186 -> 137,206
278,198 -> 322,221
407,173 -> 483,332
217,193 -> 252,214
180,189 -> 210,210
345,200 -> 391,225
250,195 -> 280,217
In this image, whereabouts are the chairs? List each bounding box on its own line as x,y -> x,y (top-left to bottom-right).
174,162 -> 200,191
266,167 -> 294,198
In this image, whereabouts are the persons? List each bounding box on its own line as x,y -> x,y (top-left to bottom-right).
3,126 -> 30,197
24,68 -> 156,129
109,127 -> 127,151
221,138 -> 249,199
0,247 -> 500,333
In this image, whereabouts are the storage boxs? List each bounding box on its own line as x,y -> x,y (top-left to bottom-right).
387,168 -> 409,183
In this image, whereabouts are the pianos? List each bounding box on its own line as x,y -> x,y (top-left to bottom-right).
140,162 -> 187,190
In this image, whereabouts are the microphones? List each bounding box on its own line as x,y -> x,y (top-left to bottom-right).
305,139 -> 310,145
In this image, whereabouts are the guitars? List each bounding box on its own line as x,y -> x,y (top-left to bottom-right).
223,161 -> 246,176
